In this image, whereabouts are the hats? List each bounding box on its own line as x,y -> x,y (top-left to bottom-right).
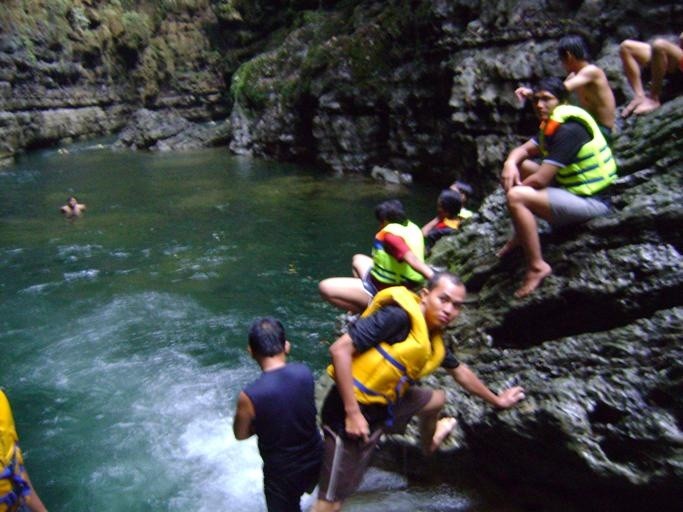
532,77 -> 570,102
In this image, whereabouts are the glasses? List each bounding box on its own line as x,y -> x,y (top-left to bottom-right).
528,94 -> 556,103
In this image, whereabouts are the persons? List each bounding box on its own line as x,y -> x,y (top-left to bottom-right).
496,76 -> 617,299
309,271 -> 525,512
317,199 -> 445,339
234,317 -> 324,512
421,179 -> 481,259
59,195 -> 87,217
0,386 -> 47,511
513,36 -> 616,139
619,35 -> 681,118
